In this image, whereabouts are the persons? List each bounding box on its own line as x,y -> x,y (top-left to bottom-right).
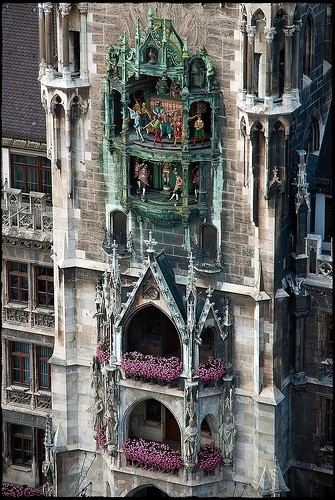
127,100 -> 205,148
128,159 -> 201,202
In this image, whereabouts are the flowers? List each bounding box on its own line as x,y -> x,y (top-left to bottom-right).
96,338 -> 226,383
95,426 -> 223,471
